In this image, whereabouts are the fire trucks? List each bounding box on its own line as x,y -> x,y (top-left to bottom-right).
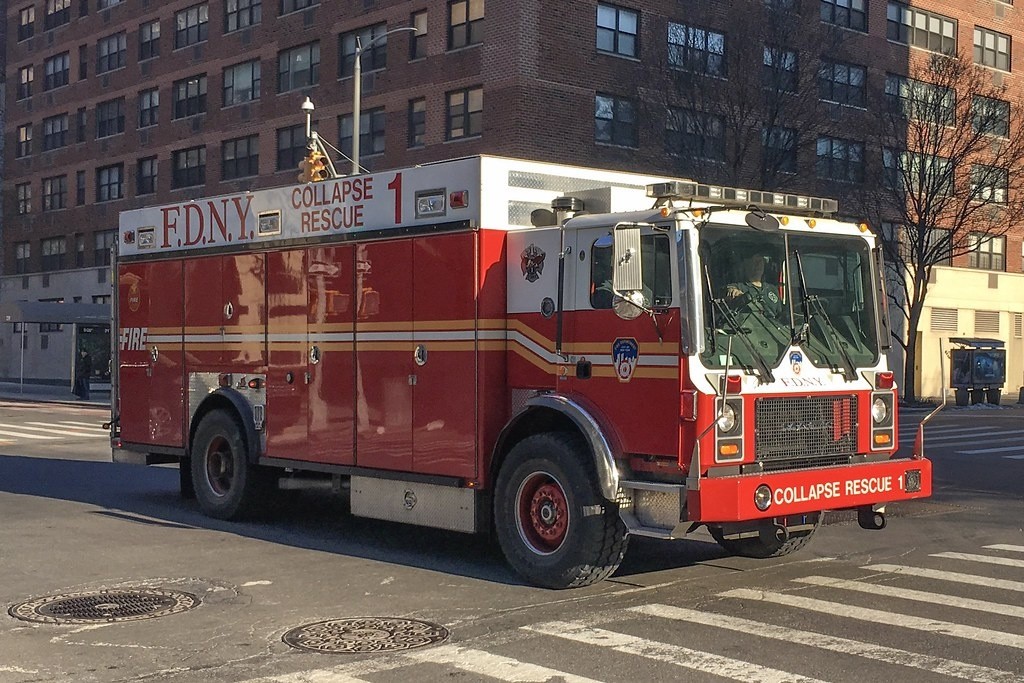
101,154 -> 947,590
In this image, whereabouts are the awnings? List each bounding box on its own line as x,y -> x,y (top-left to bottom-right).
1,302 -> 111,326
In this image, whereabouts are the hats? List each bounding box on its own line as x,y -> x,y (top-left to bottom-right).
740,246 -> 760,259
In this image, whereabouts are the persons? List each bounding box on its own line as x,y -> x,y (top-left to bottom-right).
73,347 -> 94,401
720,250 -> 784,327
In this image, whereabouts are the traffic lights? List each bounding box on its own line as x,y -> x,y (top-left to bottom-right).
312,156 -> 328,182
297,161 -> 304,182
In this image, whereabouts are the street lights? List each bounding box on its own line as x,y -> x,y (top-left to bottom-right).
349,27 -> 419,177
